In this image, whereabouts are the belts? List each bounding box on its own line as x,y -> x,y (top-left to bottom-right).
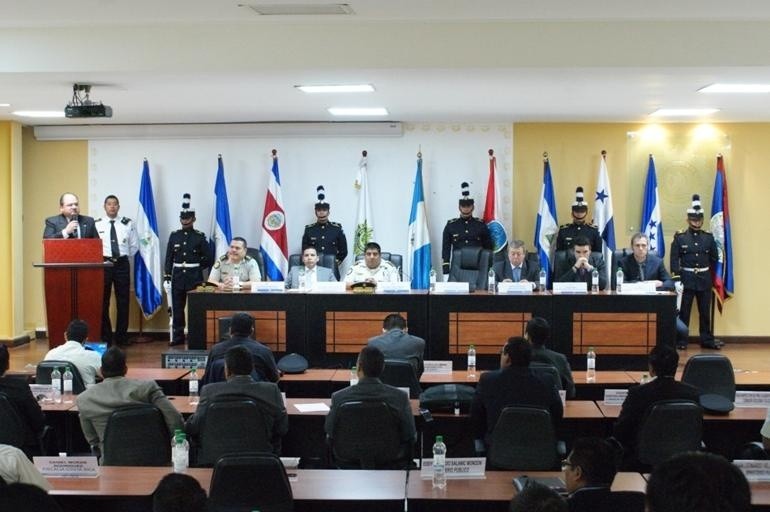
104,256 -> 129,262
173,263 -> 200,268
680,267 -> 709,272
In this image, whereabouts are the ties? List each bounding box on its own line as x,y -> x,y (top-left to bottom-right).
513,268 -> 520,282
638,264 -> 644,280
306,271 -> 312,287
109,221 -> 120,260
580,268 -> 585,280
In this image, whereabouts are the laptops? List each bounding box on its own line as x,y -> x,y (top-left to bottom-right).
83,342 -> 108,381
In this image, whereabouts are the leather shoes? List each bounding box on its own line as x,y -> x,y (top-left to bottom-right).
700,343 -> 720,349
114,339 -> 132,345
168,339 -> 185,346
679,344 -> 686,349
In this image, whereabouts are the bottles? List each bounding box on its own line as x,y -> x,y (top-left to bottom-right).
539,267 -> 546,293
50,364 -> 75,403
586,345 -> 595,380
591,267 -> 599,294
298,267 -> 306,292
616,267 -> 624,294
428,266 -> 437,292
233,266 -> 240,293
432,435 -> 448,489
170,429 -> 191,475
350,367 -> 359,385
188,368 -> 200,405
487,267 -> 495,294
640,374 -> 650,385
468,344 -> 477,378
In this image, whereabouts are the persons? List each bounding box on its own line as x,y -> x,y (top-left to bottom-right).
471,315 -> 753,511
302,200 -> 348,281
43,314 -> 188,447
442,196 -> 493,282
324,313 -> 425,465
95,195 -> 139,350
491,239 -> 540,291
617,232 -> 689,342
163,208 -> 212,349
285,246 -> 338,289
670,209 -> 720,350
207,237 -> 261,290
43,192 -> 100,239
557,235 -> 607,290
557,201 -> 602,252
152,472 -> 208,511
183,315 -> 287,456
343,243 -> 401,283
2,342 -> 50,511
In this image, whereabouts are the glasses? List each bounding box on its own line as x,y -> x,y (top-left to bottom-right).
560,459 -> 576,471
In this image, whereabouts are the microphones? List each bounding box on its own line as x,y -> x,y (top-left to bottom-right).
164,368 -> 196,393
71,211 -> 77,233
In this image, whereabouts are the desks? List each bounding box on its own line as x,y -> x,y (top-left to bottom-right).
188,283 -> 677,371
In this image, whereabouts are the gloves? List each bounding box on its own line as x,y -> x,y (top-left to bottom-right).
443,274 -> 449,281
164,281 -> 172,294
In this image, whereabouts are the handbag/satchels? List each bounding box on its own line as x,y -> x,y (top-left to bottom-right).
420,384 -> 476,412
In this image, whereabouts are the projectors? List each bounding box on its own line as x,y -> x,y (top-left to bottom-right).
64,84 -> 112,117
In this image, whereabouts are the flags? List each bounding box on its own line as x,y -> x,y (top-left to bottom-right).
258,157 -> 289,282
133,159 -> 163,321
481,156 -> 508,256
640,154 -> 665,261
533,157 -> 560,290
407,159 -> 431,289
710,156 -> 734,315
207,158 -> 232,265
592,154 -> 616,290
353,158 -> 374,265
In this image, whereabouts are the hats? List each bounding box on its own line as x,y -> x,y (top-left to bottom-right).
700,394 -> 733,415
278,353 -> 308,374
351,282 -> 376,295
459,182 -> 473,207
315,186 -> 329,210
179,194 -> 195,220
687,194 -> 704,216
572,187 -> 587,212
